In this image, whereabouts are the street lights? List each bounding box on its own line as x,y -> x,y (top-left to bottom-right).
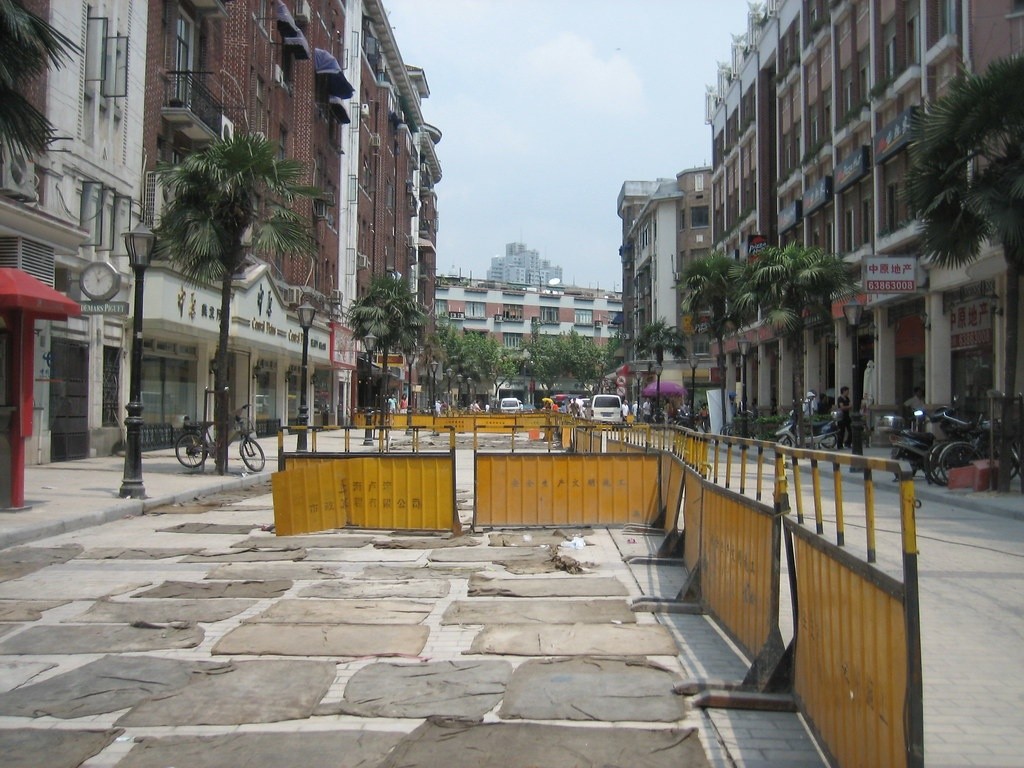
688,353 -> 699,429
119,218 -> 158,499
294,300 -> 317,453
456,373 -> 464,411
736,334 -> 752,450
842,295 -> 867,474
404,349 -> 417,431
362,330 -> 380,446
445,367 -> 453,418
653,363 -> 664,432
634,369 -> 643,424
466,377 -> 472,415
429,361 -> 439,436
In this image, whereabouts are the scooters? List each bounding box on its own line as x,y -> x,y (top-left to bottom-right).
774,408 -> 844,459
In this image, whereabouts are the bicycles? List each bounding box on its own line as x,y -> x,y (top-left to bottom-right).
174,402 -> 266,473
876,392 -> 1023,489
672,408 -> 759,446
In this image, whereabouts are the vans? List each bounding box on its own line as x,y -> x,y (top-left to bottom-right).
497,397 -> 520,415
584,394 -> 624,431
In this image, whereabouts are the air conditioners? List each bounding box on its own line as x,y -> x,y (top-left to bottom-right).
275,64 -> 283,87
373,133 -> 380,149
0,132 -> 34,201
449,312 -> 629,339
332,290 -> 343,305
358,254 -> 368,270
145,171 -> 177,229
317,202 -> 328,220
397,110 -> 426,249
285,288 -> 301,305
378,59 -> 385,73
295,0 -> 311,25
361,103 -> 370,116
216,113 -> 233,146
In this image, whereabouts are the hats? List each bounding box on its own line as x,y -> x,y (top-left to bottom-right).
806,391 -> 816,398
624,400 -> 629,405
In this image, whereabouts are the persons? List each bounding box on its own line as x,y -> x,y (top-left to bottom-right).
435,400 -> 447,412
903,387 -> 924,413
803,390 -> 817,415
470,400 -> 481,412
388,393 -> 407,413
837,387 -> 852,449
545,398 -> 580,417
770,398 -> 776,416
958,384 -> 977,421
817,393 -> 832,414
622,397 -> 708,423
752,399 -> 758,416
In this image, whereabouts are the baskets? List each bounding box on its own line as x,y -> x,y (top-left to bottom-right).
235,414 -> 256,433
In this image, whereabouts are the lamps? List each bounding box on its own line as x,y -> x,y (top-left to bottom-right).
208,358 -> 219,375
310,374 -> 317,384
286,367 -> 292,382
253,364 -> 261,379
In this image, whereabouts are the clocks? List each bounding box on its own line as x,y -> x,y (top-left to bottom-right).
79,262 -> 120,301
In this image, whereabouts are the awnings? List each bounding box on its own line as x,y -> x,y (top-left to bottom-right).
611,315 -> 623,324
315,49 -> 355,99
329,96 -> 351,125
276,0 -> 309,60
419,237 -> 436,253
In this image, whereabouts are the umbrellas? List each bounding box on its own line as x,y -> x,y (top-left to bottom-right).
643,380 -> 688,395
542,398 -> 553,403
